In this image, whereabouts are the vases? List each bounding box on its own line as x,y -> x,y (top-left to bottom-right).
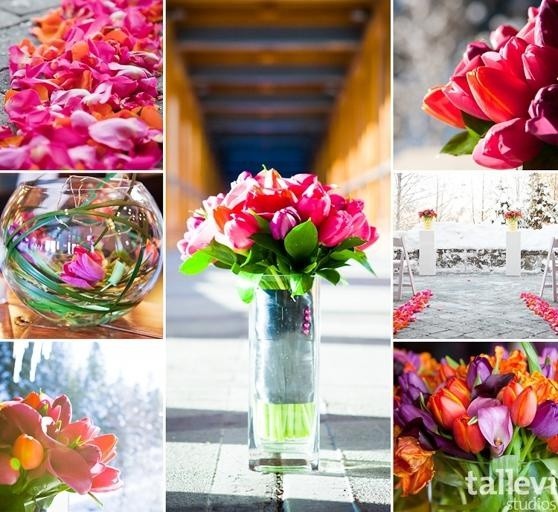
248,288 -> 320,474
430,453 -> 538,510
510,219 -> 518,229
424,218 -> 432,231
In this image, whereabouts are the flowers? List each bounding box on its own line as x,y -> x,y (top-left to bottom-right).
393,342 -> 558,504
418,209 -> 441,222
0,391 -> 122,512
174,162 -> 383,304
420,0 -> 558,170
504,210 -> 524,220
